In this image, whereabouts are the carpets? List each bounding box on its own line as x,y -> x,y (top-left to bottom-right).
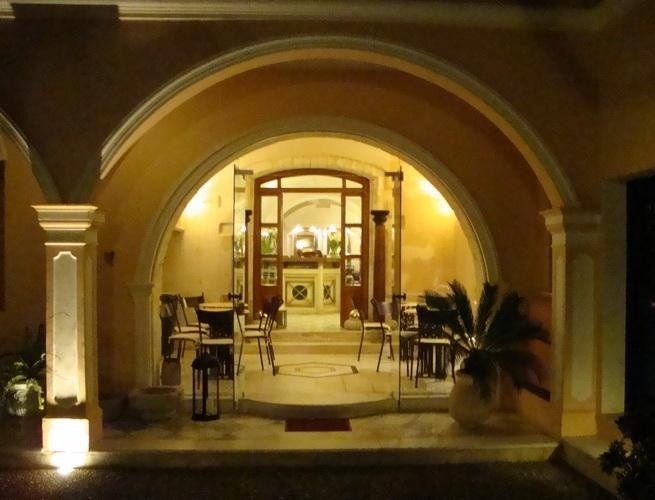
283,417 -> 352,433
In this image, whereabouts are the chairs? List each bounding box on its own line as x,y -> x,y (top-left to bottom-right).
158,291 -> 284,390
352,293 -> 460,389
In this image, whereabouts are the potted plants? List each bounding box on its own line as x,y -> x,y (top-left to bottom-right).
417,278 -> 551,430
0,347 -> 46,450
